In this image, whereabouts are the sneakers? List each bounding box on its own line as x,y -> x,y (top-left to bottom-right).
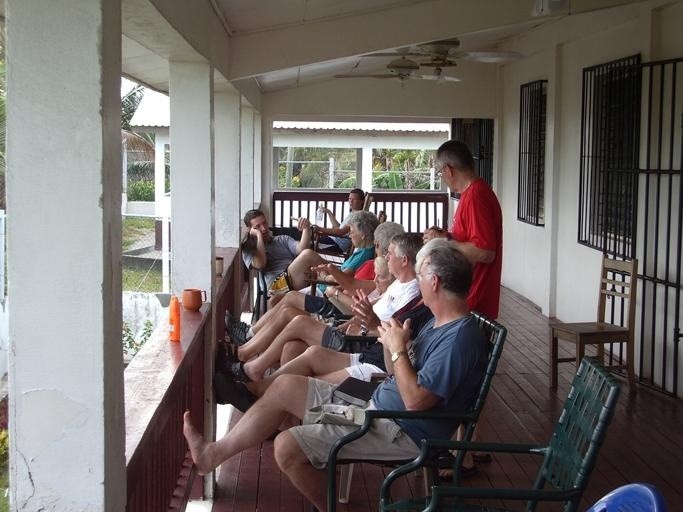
225,317 -> 246,346
226,310 -> 246,331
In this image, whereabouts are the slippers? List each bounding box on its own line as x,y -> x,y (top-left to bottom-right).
217,337 -> 244,363
213,373 -> 258,414
217,355 -> 251,383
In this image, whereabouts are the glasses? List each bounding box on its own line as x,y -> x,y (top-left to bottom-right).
436,164 -> 448,177
373,241 -> 379,249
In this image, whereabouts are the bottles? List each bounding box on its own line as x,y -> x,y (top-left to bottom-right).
216,257 -> 224,277
169,296 -> 181,341
317,202 -> 323,220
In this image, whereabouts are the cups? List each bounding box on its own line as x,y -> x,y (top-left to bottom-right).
181,287 -> 207,313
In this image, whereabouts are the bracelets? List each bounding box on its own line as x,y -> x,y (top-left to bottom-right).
335,289 -> 338,300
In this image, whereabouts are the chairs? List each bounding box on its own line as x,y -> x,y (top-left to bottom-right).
382,357 -> 621,511
254,227 -> 316,322
326,310 -> 506,512
316,192 -> 370,269
549,255 -> 638,398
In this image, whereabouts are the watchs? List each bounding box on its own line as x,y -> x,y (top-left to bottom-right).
390,351 -> 407,364
360,326 -> 368,336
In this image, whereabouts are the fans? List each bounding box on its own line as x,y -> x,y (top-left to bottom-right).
358,38 -> 519,67
334,54 -> 424,87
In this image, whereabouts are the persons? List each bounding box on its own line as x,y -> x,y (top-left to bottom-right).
421,140 -> 503,476
242,208 -> 336,299
223,222 -> 404,347
269,188 -> 367,255
268,210 -> 379,310
216,231 -> 423,383
218,254 -> 396,363
212,236 -> 466,413
182,248 -> 489,511
